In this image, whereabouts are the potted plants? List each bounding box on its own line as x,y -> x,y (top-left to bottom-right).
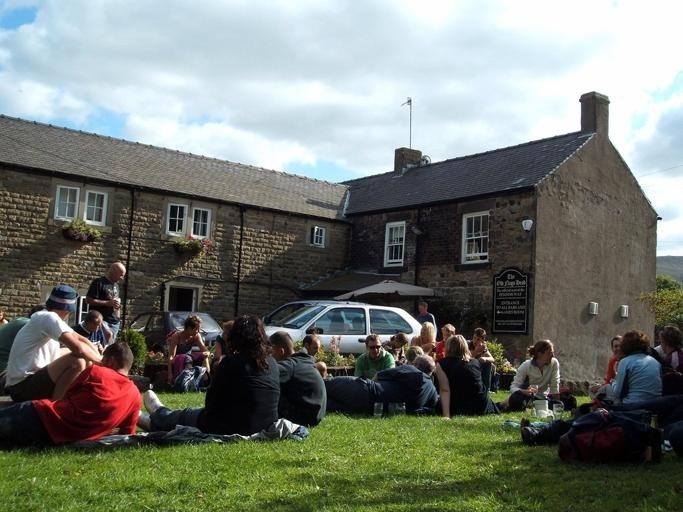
169,234 -> 212,254
61,219 -> 101,244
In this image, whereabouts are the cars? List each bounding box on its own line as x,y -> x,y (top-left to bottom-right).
263,304 -> 424,361
125,310 -> 223,356
259,300 -> 371,329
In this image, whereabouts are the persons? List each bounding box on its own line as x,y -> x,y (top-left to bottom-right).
417,302 -> 435,326
1,286 -> 143,448
163,316 -> 327,427
135,313 -> 279,432
302,321 -> 500,419
522,322 -> 683,464
86,262 -> 126,339
495,339 -> 577,412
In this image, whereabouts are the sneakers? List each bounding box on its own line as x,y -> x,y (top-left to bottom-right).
521,417 -> 544,446
144,389 -> 164,415
135,410 -> 151,431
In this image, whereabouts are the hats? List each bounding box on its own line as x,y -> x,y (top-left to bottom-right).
46,284 -> 79,312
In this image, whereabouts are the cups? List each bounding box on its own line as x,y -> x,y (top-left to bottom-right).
374,402 -> 383,416
534,400 -> 548,411
552,403 -> 563,420
113,297 -> 121,310
529,384 -> 539,395
537,409 -> 547,418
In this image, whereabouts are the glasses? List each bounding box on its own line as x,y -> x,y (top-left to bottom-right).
367,345 -> 382,349
